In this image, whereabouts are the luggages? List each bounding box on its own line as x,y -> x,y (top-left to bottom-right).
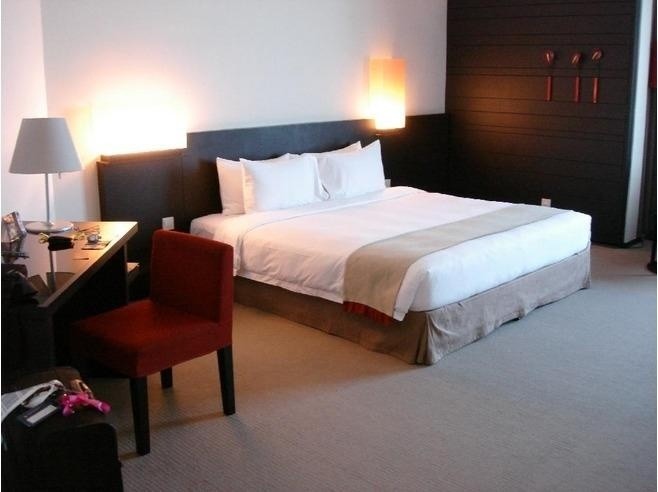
1,365 -> 124,492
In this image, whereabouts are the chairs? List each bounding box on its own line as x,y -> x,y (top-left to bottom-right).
67,228 -> 237,457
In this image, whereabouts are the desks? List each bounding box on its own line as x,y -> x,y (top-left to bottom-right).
1,219 -> 139,363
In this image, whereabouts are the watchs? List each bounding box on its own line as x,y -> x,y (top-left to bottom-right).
86,234 -> 102,244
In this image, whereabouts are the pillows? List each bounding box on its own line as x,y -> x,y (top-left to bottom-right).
215,140 -> 386,217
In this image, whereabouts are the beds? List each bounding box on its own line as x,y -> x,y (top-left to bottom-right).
185,118 -> 594,366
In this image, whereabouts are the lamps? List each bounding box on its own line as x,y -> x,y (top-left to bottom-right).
8,116 -> 84,232
